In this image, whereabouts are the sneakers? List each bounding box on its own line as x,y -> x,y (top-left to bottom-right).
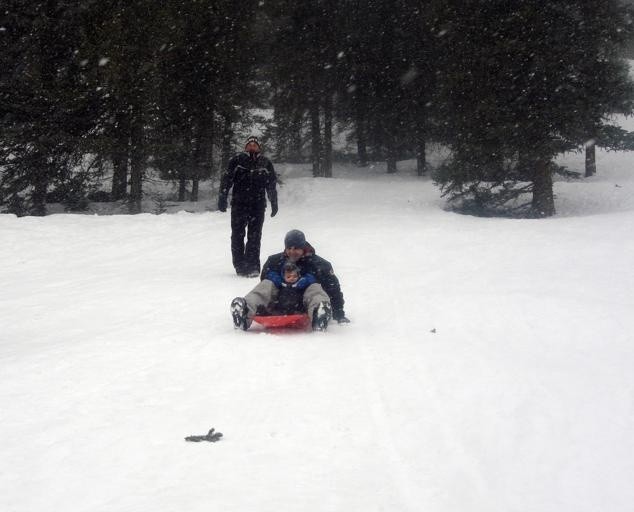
230,296 -> 249,330
312,301 -> 332,332
237,270 -> 259,276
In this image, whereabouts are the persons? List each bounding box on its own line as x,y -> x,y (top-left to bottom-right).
217,135 -> 277,278
229,228 -> 350,332
267,262 -> 316,312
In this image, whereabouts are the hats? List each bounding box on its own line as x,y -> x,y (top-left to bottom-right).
284,229 -> 306,248
244,135 -> 262,152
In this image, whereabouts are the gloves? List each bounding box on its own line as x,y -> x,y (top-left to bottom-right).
337,317 -> 350,323
218,200 -> 228,212
270,204 -> 278,217
297,273 -> 317,290
265,269 -> 284,288
